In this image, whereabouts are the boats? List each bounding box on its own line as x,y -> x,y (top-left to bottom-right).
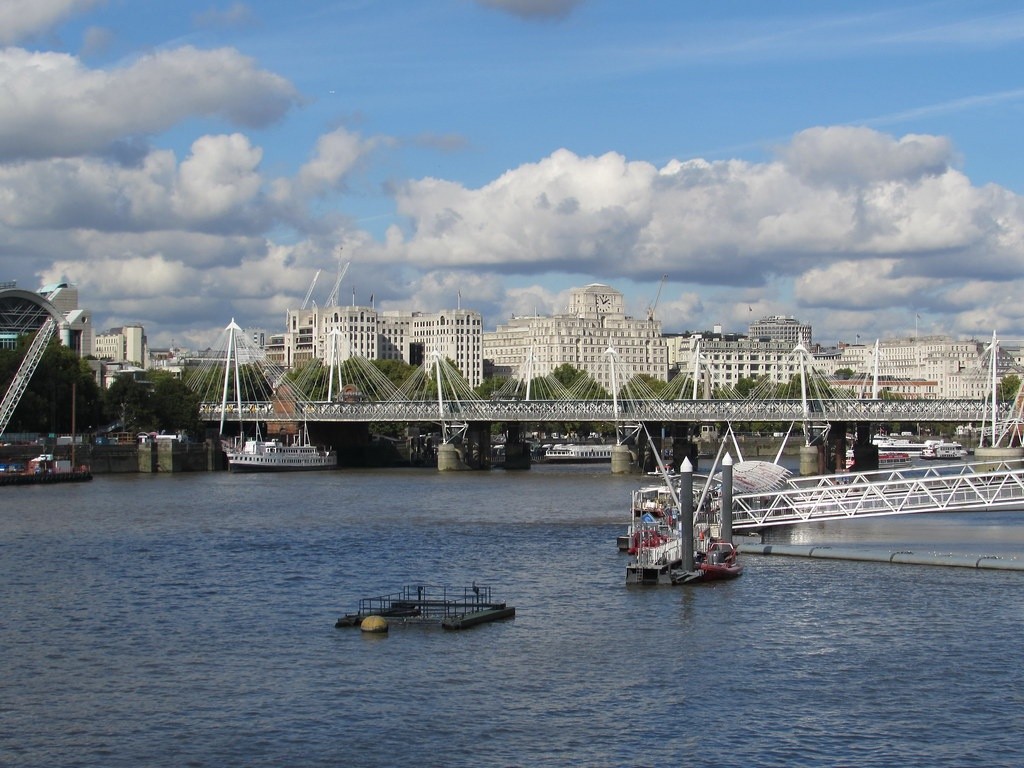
920,442 -> 968,460
545,445 -> 612,463
869,435 -> 891,446
701,543 -> 742,576
225,405 -> 337,473
877,439 -> 928,451
845,449 -> 912,469
647,464 -> 675,477
